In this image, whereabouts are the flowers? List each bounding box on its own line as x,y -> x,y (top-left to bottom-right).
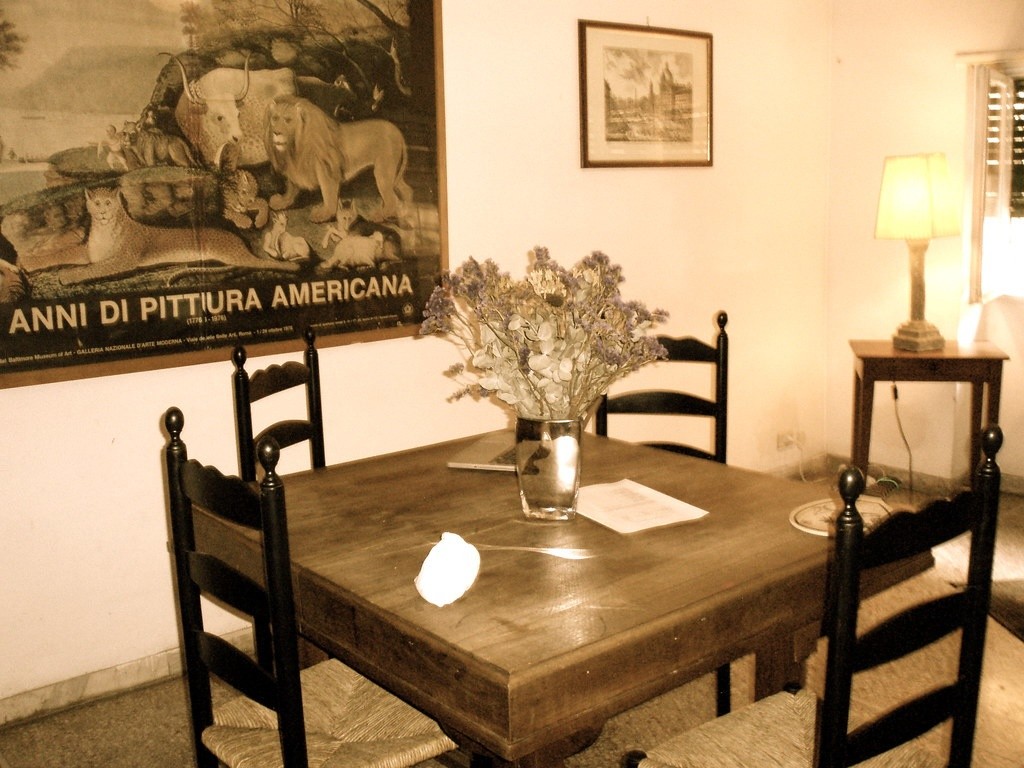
418,247 -> 669,420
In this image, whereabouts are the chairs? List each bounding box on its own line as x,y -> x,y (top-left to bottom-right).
595,310 -> 727,466
163,406 -> 459,768
619,418 -> 1003,768
232,326 -> 325,668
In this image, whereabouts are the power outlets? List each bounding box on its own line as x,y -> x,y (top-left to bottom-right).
777,429 -> 806,450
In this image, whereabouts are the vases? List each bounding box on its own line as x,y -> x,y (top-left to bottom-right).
516,415 -> 585,521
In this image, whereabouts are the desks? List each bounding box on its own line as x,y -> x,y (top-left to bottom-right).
847,338 -> 1011,492
193,427 -> 937,768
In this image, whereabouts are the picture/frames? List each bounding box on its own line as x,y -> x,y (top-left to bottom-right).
577,18 -> 714,169
0,0 -> 455,391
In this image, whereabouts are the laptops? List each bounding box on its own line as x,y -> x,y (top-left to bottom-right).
445,395 -> 603,472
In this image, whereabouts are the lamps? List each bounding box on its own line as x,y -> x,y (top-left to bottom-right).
874,150 -> 963,353
